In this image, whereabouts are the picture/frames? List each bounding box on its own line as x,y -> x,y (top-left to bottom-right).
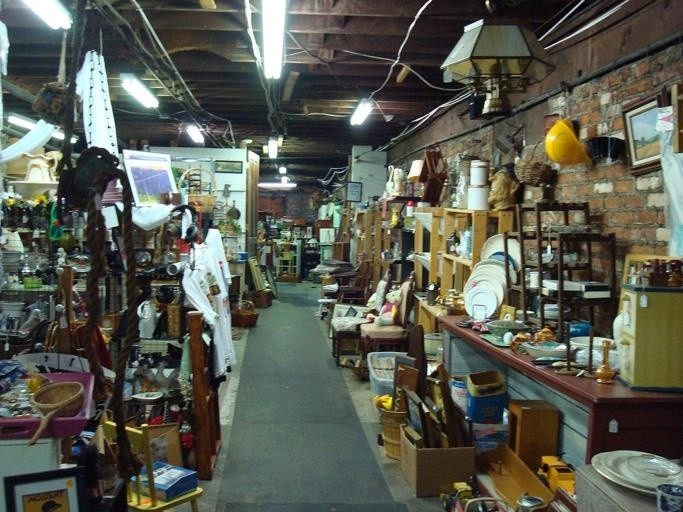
3,465 -> 90,512
621,86 -> 671,175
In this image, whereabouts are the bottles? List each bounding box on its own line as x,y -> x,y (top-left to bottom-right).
594,340 -> 616,384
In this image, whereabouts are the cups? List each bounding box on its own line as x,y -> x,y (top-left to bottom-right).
656,484 -> 683,512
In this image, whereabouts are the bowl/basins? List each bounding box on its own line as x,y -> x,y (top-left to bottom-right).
587,136 -> 626,165
485,319 -> 531,341
521,335 -> 621,372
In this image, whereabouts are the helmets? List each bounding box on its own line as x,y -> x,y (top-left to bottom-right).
544,119 -> 593,167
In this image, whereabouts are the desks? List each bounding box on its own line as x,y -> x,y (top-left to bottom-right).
439,308 -> 683,472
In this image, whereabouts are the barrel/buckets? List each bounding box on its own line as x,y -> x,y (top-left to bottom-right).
131,359 -> 180,394
468,185 -> 490,211
2,250 -> 23,272
470,160 -> 489,185
0,303 -> 25,316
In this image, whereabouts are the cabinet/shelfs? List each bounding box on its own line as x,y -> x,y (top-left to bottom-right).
0,178 -> 223,405
360,196 -> 517,336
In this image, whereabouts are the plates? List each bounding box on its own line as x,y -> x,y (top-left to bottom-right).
591,450 -> 683,498
463,234 -> 521,319
543,304 -> 571,320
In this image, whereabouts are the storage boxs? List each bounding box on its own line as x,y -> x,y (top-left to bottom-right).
399,423 -> 478,500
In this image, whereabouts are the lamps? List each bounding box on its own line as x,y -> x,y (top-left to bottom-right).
437,24 -> 535,119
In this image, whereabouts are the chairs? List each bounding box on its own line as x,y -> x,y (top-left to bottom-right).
317,255 -> 416,373
103,412 -> 205,512
